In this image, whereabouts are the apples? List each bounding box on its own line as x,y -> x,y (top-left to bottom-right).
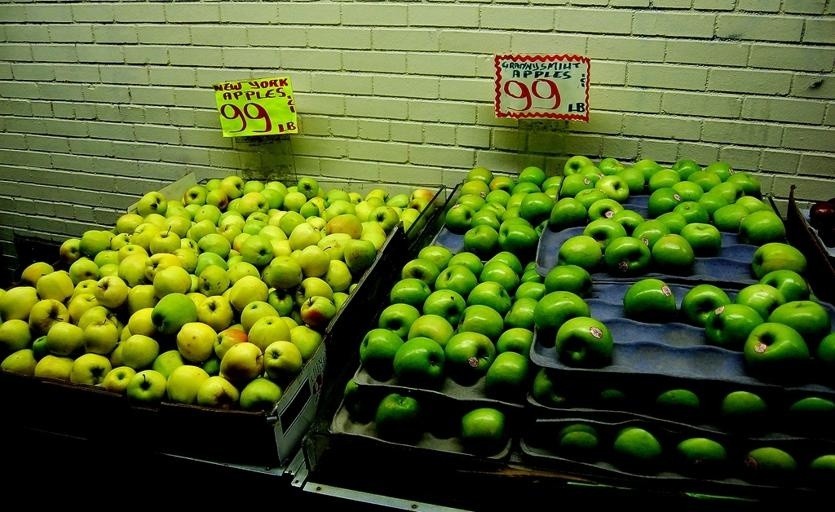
0,154 -> 835,487
